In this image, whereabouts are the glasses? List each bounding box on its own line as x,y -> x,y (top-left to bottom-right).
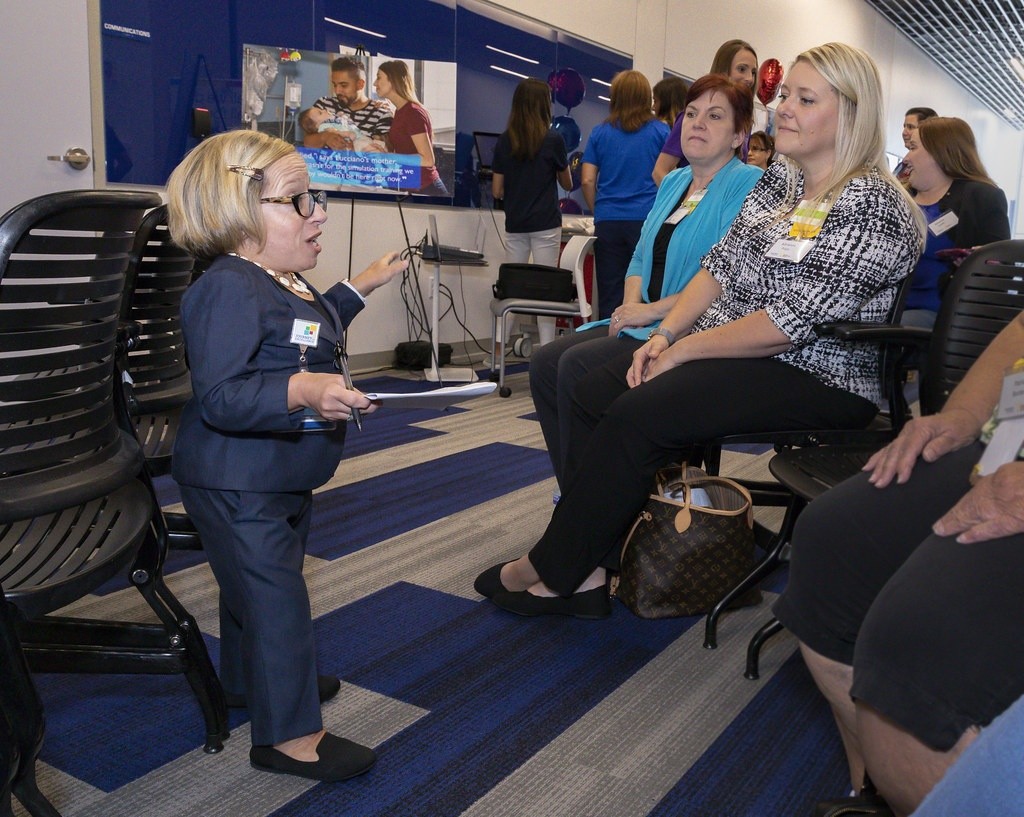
751,146 -> 766,154
259,188 -> 328,218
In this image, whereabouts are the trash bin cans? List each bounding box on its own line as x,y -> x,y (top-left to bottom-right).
555,233 -> 594,329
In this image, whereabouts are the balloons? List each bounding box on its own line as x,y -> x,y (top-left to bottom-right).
549,68 -> 585,114
755,58 -> 784,107
569,153 -> 583,192
553,117 -> 582,153
560,198 -> 584,215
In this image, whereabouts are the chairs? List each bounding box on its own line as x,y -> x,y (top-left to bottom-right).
0,187 -> 231,817
684,237 -> 1024,684
489,235 -> 598,397
11,204 -> 206,552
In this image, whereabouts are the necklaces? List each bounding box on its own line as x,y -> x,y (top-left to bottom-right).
228,252 -> 311,295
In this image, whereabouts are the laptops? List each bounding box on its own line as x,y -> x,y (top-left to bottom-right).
472,131 -> 502,175
429,214 -> 488,264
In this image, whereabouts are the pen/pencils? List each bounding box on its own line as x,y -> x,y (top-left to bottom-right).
335,340 -> 362,433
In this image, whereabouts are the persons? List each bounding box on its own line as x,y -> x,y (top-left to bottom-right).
652,77 -> 689,128
164,127 -> 408,779
473,41 -> 929,619
528,72 -> 766,495
582,68 -> 671,321
374,60 -> 449,195
483,78 -> 574,369
652,38 -> 758,186
771,306 -> 1024,817
893,109 -> 1014,311
746,131 -> 776,169
298,107 -> 397,175
303,56 -> 393,187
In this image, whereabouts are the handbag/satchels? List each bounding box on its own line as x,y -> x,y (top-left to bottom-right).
813,767 -> 897,817
609,458 -> 764,619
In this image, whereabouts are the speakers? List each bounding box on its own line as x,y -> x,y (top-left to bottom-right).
191,108 -> 211,139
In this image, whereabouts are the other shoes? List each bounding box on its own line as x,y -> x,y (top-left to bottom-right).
473,556 -> 524,598
225,668 -> 340,709
482,356 -> 501,370
499,578 -> 611,620
250,726 -> 378,781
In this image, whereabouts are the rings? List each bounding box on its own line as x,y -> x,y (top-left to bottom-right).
615,315 -> 619,323
346,413 -> 352,421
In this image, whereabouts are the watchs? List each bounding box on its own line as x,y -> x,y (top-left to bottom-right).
648,328 -> 676,346
